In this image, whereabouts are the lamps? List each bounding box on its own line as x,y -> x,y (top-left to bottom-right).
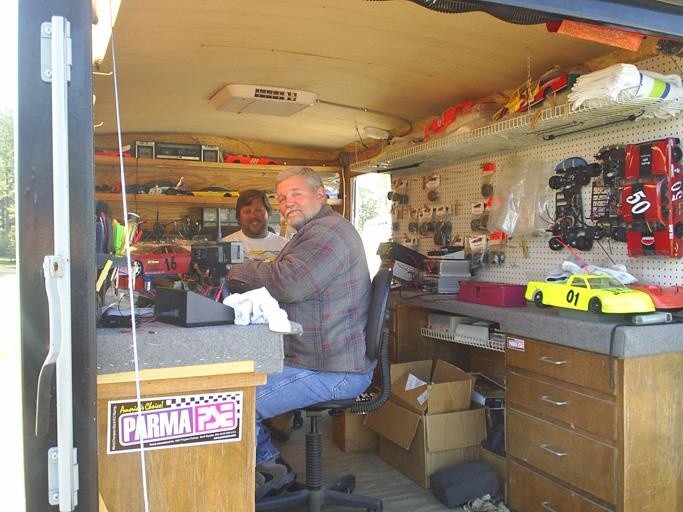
93,1 -> 122,68
363,127 -> 390,140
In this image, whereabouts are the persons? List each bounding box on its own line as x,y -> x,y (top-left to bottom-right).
221,190 -> 290,262
225,166 -> 379,502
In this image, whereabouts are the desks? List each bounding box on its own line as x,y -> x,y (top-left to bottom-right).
371,286 -> 683,512
96,320 -> 284,512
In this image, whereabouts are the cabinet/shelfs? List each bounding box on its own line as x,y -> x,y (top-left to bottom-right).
95,155 -> 345,247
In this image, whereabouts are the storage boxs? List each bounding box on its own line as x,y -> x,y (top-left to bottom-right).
333,358 -> 486,490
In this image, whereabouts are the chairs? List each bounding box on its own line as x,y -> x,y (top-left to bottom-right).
255,265 -> 394,512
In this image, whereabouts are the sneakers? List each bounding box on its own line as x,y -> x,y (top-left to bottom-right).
255,453 -> 297,503
